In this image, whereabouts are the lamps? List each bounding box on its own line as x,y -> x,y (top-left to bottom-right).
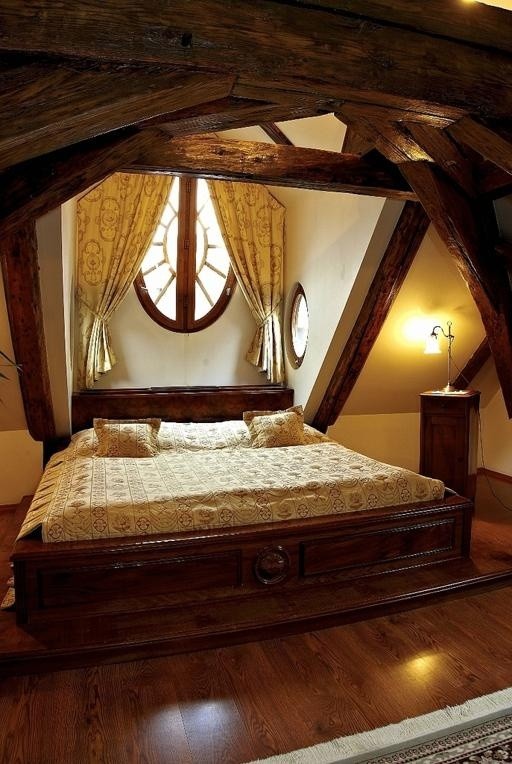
423,320 -> 473,395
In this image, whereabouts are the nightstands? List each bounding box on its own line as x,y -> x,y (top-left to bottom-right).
418,389 -> 481,501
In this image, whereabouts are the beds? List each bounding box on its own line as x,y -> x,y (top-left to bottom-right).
9,383 -> 476,626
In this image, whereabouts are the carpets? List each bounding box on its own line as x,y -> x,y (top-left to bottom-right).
245,687 -> 512,764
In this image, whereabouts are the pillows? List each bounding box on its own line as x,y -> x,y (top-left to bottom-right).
243,405 -> 312,448
92,418 -> 161,459
